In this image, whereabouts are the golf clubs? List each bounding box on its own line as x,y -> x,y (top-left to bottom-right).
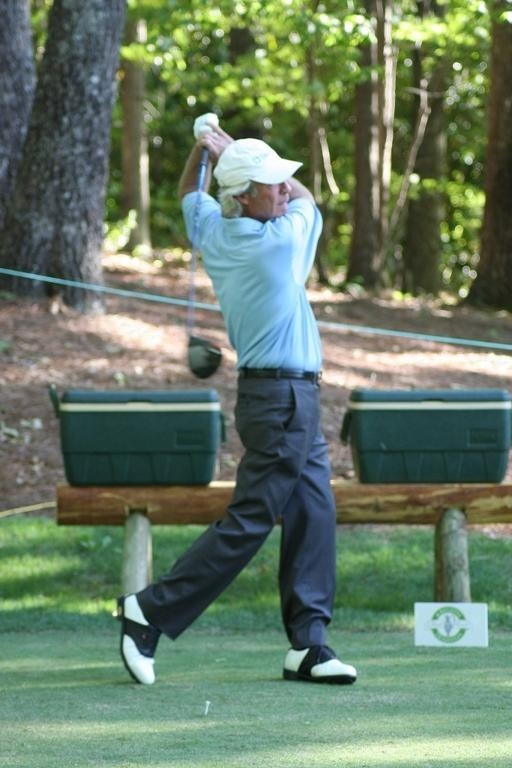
187,148 -> 222,378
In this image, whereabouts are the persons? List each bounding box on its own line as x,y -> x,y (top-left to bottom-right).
114,109 -> 359,684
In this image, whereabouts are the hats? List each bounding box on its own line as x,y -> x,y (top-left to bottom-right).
212,138 -> 304,187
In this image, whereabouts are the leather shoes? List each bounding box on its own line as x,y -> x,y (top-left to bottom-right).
282,645 -> 357,686
117,592 -> 164,686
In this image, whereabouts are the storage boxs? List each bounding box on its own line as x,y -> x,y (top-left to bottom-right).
44,378 -> 227,487
337,382 -> 512,484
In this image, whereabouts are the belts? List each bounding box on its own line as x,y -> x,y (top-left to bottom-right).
235,367 -> 324,381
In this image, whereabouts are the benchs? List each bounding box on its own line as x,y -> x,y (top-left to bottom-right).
53,481 -> 512,625
